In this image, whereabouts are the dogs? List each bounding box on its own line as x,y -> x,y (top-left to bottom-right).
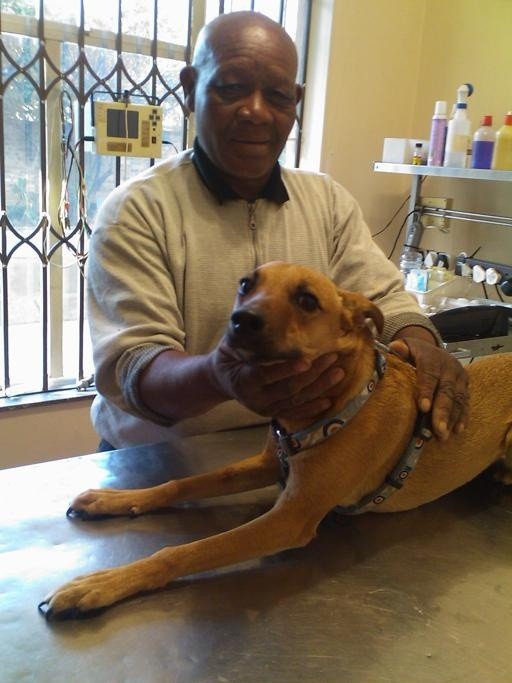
32,259 -> 512,624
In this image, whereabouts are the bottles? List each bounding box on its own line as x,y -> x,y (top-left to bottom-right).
490,112 -> 511,169
412,142 -> 423,165
399,249 -> 422,285
426,100 -> 447,167
470,115 -> 497,169
443,102 -> 474,168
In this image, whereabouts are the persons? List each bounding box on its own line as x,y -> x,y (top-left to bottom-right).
85,11 -> 469,452
